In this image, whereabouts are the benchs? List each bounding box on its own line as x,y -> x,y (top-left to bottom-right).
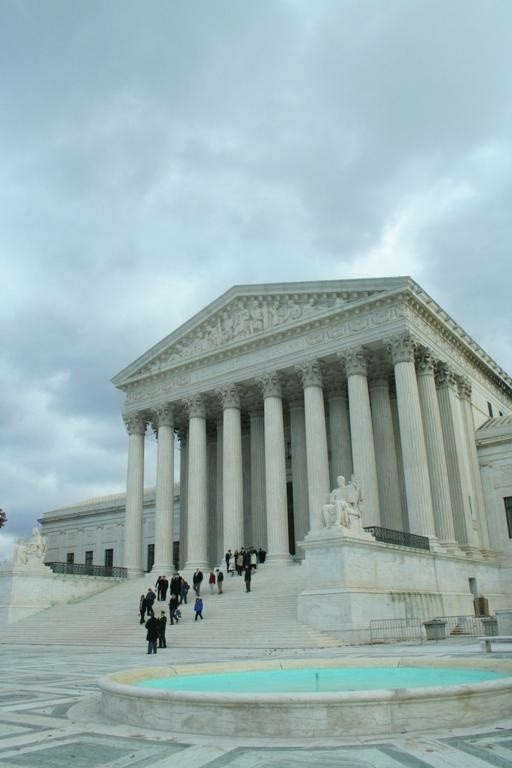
477,636 -> 512,653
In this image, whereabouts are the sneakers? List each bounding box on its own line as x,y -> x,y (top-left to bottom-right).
169,618 -> 178,625
217,592 -> 223,594
146,645 -> 166,654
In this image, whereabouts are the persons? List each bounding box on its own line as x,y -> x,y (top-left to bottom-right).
20,527 -> 42,564
139,547 -> 265,654
322,476 -> 356,528
